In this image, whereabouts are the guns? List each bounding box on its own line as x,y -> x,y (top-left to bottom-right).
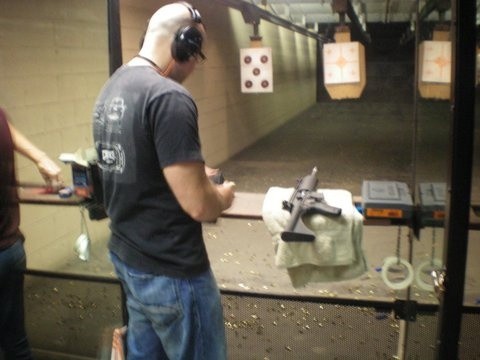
280,166 -> 341,242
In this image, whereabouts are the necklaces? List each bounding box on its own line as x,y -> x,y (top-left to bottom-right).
137,55 -> 168,79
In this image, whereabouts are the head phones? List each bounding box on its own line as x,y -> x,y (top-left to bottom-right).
139,2 -> 203,63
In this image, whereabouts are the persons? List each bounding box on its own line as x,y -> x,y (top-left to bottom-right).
93,0 -> 237,360
0,105 -> 64,359
